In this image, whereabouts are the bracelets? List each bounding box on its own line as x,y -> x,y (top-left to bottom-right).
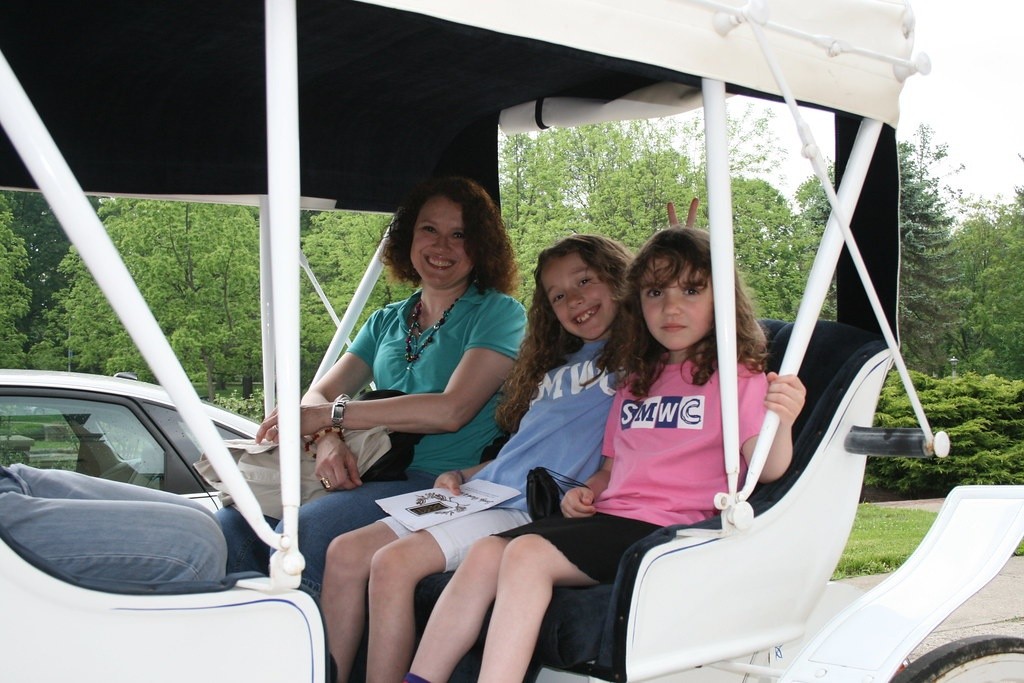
455,470 -> 465,484
405,297 -> 458,361
331,401 -> 346,428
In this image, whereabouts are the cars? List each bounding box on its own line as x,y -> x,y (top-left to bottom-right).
0,364 -> 275,518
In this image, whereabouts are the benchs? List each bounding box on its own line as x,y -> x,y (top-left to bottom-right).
0,532 -> 328,683
415,320 -> 900,682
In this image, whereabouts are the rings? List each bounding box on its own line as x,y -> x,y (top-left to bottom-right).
273,425 -> 278,430
320,478 -> 330,488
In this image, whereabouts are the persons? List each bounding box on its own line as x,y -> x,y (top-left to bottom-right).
402,228 -> 807,683
319,198 -> 699,683
217,178 -> 527,602
0,462 -> 227,584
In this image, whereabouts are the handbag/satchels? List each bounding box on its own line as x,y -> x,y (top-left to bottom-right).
353,389 -> 428,482
526,466 -> 591,521
